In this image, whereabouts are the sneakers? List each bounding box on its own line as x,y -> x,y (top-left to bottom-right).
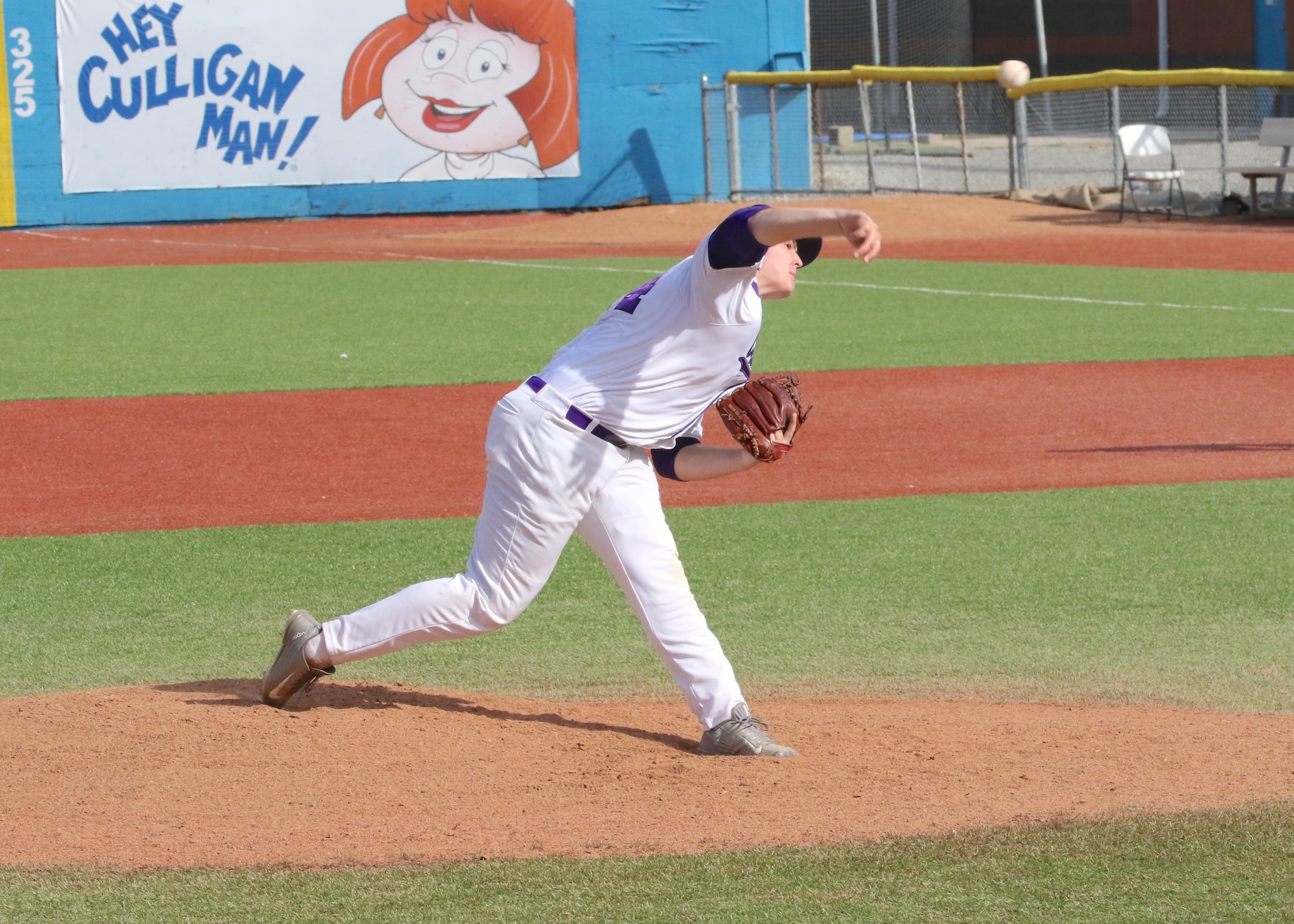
260,608 -> 335,709
697,702 -> 799,756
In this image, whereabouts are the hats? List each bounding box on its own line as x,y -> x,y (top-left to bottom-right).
796,237 -> 824,268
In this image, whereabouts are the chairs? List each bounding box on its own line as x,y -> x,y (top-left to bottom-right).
1116,123 -> 1189,223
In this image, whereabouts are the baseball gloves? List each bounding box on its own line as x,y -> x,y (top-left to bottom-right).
715,373 -> 805,463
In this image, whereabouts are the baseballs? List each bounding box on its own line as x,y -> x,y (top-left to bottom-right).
998,59 -> 1029,88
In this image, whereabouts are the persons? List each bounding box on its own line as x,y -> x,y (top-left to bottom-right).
263,202 -> 882,757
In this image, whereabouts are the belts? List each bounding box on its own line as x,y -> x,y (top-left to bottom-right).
526,375 -> 628,449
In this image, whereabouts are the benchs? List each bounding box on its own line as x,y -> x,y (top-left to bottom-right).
1219,116 -> 1294,219
